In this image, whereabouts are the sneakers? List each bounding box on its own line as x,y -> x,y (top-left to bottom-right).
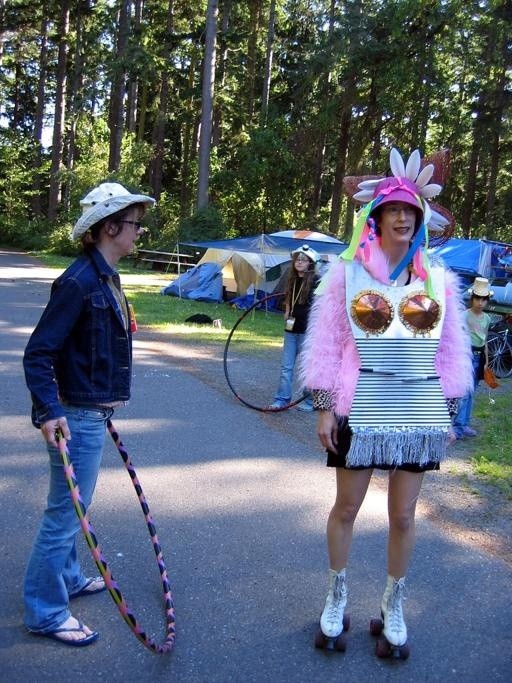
297,403 -> 316,412
267,402 -> 291,410
453,426 -> 477,440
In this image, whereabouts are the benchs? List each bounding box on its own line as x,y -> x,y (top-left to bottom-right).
142,258 -> 196,273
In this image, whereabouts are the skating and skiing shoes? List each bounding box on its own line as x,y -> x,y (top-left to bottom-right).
315,569 -> 350,652
369,573 -> 411,660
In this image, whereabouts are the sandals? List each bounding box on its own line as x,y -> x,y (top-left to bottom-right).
68,576 -> 108,600
30,616 -> 99,646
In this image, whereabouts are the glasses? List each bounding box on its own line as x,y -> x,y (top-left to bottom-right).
110,216 -> 143,230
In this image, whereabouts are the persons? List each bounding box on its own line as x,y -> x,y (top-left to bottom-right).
456,277 -> 493,440
24,180 -> 157,644
268,243 -> 324,413
297,145 -> 472,648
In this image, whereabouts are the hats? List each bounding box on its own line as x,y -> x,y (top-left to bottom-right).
72,182 -> 156,243
290,244 -> 322,264
467,277 -> 494,298
369,177 -> 424,238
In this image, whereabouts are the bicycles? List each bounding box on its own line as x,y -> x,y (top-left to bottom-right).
486,314 -> 511,378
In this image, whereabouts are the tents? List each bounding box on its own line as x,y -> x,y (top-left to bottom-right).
161,228 -> 512,331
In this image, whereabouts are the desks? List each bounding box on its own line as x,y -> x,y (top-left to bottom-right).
132,249 -> 194,271
